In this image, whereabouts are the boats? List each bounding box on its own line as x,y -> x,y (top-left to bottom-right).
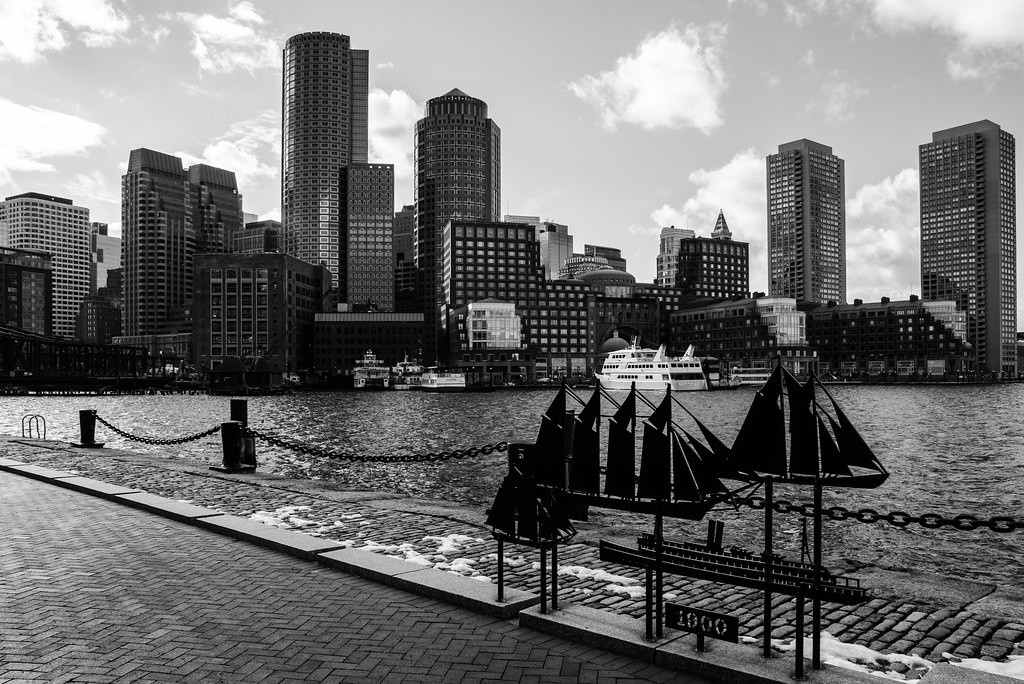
593,335 -> 742,393
349,347 -> 467,393
